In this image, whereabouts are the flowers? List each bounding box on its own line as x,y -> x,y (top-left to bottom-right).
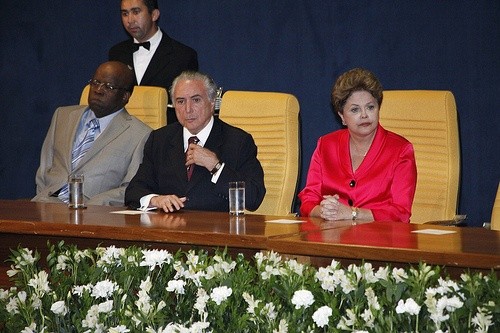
0,240 -> 500,333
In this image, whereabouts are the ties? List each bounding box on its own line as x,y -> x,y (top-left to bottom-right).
185,137 -> 200,181
57,118 -> 99,204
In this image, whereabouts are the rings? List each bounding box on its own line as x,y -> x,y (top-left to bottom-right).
189,154 -> 191,159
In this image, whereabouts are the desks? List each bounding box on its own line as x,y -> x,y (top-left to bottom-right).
0,198 -> 500,299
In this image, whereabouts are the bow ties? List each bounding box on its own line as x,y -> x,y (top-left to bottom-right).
130,42 -> 151,53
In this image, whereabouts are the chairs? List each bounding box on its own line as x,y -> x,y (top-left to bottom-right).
218,91 -> 301,216
79,83 -> 168,131
377,89 -> 460,225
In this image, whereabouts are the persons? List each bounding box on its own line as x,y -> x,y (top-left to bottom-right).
108,0 -> 200,126
31,61 -> 153,207
297,69 -> 417,222
124,72 -> 266,214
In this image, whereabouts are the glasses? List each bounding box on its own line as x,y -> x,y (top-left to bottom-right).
88,80 -> 123,92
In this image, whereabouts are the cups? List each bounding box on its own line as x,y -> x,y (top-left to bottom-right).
69,209 -> 83,224
228,181 -> 245,215
229,215 -> 245,235
68,174 -> 84,209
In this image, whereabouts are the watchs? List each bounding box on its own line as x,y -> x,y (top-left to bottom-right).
210,160 -> 222,175
351,206 -> 360,220
